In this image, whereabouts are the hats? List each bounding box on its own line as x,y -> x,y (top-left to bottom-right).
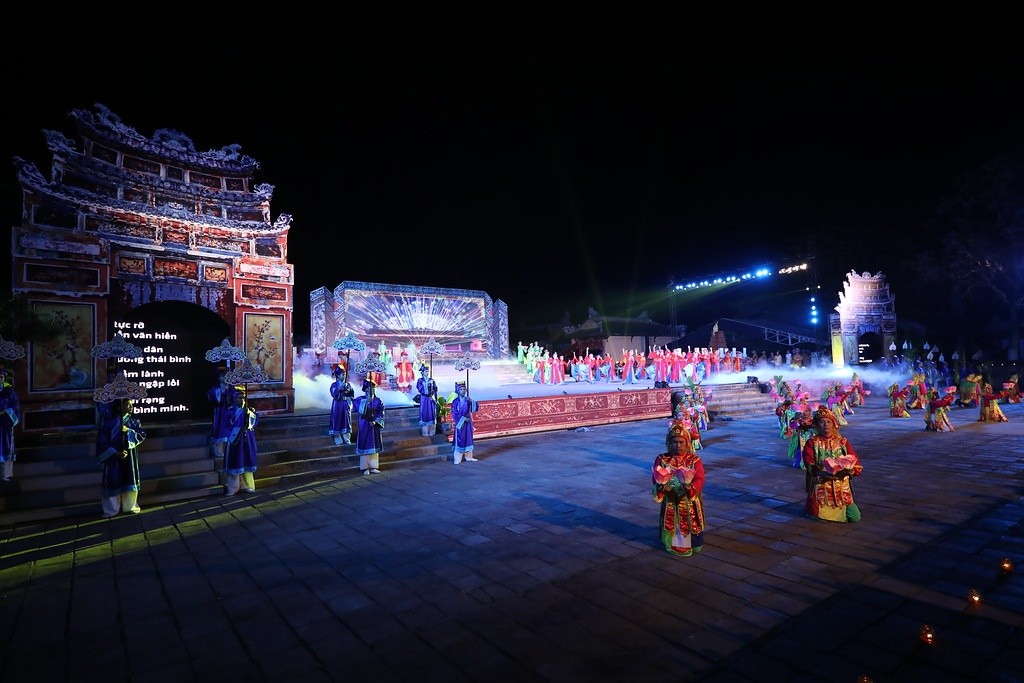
886,373 -> 983,398
984,383 -> 993,393
454,380 -> 466,394
231,385 -> 246,400
812,405 -> 839,430
419,364 -> 429,371
0,368 -> 8,377
666,422 -> 692,449
333,365 -> 345,376
362,379 -> 377,391
1010,373 -> 1018,382
217,366 -> 229,376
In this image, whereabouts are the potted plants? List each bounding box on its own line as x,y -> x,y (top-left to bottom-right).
437,392 -> 456,434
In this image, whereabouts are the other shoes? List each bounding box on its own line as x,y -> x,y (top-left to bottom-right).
336,442 -> 343,444
467,458 -> 477,461
364,470 -> 369,475
371,469 -> 380,473
430,433 -> 433,436
244,488 -> 255,493
226,492 -> 235,496
131,510 -> 140,514
424,433 -> 429,437
346,441 -> 351,445
454,460 -> 459,465
102,513 -> 111,518
216,454 -> 224,456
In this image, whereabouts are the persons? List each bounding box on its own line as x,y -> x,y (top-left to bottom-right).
329,367 -> 354,445
802,406 -> 864,523
353,379 -> 384,475
366,352 -> 382,387
408,339 -> 417,361
452,380 -> 479,465
416,365 -> 437,437
517,341 -> 1024,469
652,419 -> 705,557
378,339 -> 389,365
208,369 -> 259,495
94,369 -> 147,519
331,351 -> 347,381
395,352 -> 414,393
394,343 -> 402,359
0,368 -> 20,481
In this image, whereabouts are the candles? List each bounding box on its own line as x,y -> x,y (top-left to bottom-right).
969,588 -> 983,608
1000,556 -> 1013,573
919,624 -> 936,645
857,674 -> 873,683
